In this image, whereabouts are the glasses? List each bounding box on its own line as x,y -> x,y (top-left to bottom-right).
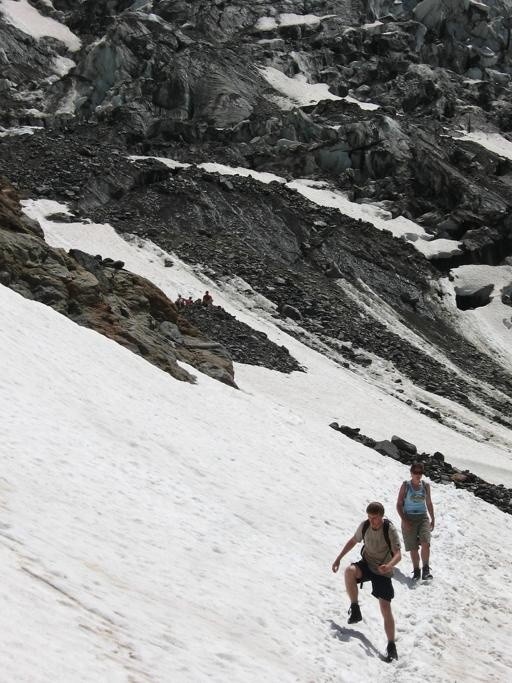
412,470 -> 424,475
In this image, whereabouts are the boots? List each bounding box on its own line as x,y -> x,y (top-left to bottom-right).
385,640 -> 399,662
347,604 -> 362,624
410,568 -> 421,581
421,565 -> 433,580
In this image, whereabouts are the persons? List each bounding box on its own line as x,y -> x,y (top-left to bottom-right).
332,501 -> 402,662
395,462 -> 436,582
